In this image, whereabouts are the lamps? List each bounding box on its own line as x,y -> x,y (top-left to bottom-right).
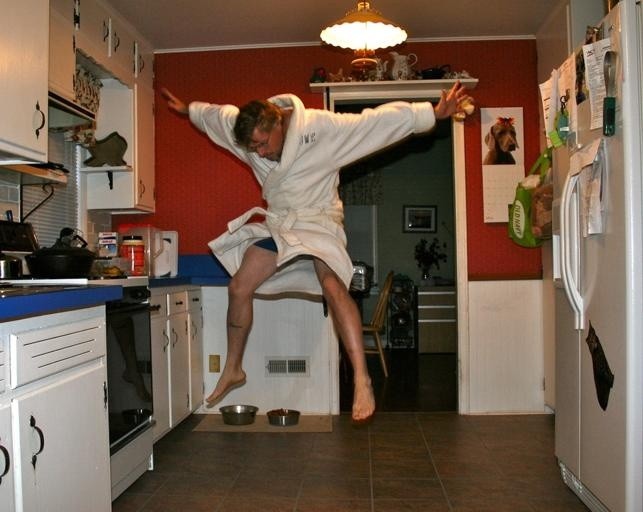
319,0 -> 408,53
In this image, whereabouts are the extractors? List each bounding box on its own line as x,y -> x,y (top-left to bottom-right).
0,165 -> 68,187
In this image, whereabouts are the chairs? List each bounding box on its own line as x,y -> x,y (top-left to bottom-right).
342,270 -> 393,382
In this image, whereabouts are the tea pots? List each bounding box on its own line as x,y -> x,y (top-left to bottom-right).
374,56 -> 388,81
390,51 -> 417,80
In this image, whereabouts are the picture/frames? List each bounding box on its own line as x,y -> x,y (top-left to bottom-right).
402,205 -> 437,233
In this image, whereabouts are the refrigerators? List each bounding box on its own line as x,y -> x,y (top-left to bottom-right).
551,0 -> 643,486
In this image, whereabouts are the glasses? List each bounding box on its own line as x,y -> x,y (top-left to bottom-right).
246,133 -> 270,152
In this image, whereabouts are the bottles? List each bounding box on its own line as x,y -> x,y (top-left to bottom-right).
119,236 -> 145,275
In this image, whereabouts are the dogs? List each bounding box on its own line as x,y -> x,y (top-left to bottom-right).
483,122 -> 520,164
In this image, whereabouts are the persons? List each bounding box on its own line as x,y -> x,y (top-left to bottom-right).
159,75 -> 470,422
112,318 -> 152,405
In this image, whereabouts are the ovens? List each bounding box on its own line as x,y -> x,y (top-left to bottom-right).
108,284 -> 157,455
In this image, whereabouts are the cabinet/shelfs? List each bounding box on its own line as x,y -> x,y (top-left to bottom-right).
1,304 -> 112,512
85,34 -> 156,217
48,1 -> 136,121
149,290 -> 204,441
0,0 -> 50,164
386,278 -> 419,352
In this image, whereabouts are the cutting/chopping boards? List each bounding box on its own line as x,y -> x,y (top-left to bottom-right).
154,230 -> 179,277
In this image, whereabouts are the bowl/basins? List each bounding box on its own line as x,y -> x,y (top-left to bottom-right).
266,409 -> 300,426
219,405 -> 259,425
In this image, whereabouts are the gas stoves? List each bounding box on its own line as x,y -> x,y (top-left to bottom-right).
0,273 -> 127,281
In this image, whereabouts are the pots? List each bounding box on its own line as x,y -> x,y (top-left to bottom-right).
0,251 -> 23,279
23,246 -> 95,280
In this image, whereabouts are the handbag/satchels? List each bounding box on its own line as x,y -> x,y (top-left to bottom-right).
509,188 -> 543,248
